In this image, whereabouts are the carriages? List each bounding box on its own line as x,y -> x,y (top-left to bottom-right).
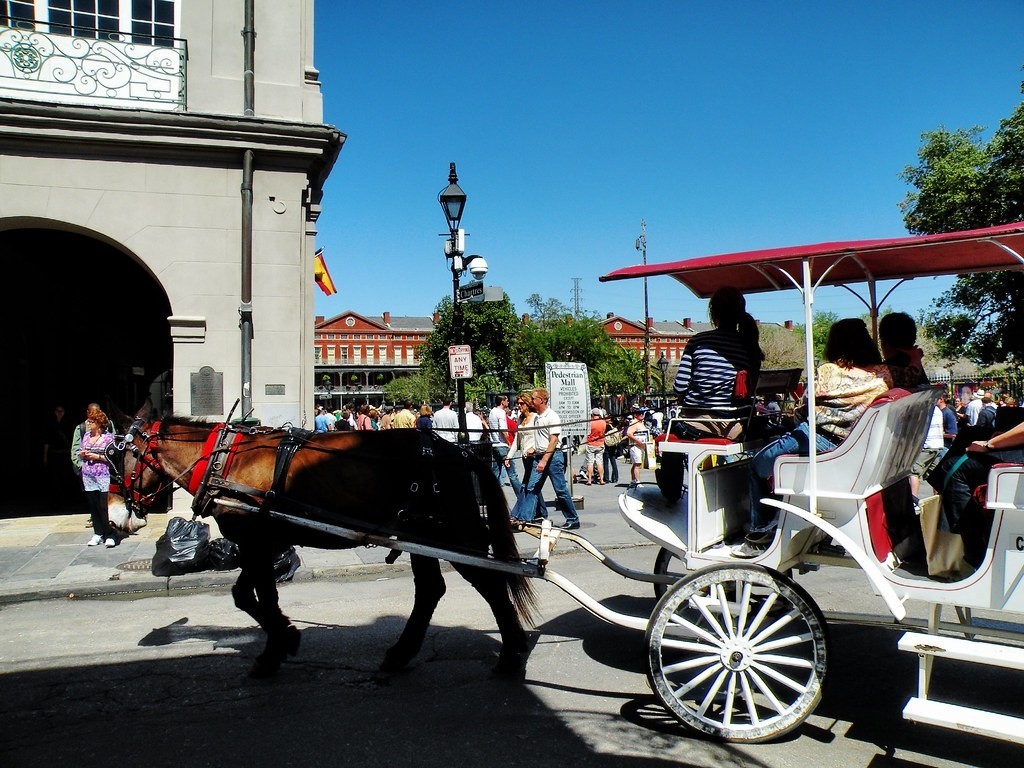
108,218 -> 1024,750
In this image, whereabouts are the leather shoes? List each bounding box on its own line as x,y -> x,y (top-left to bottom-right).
560,521 -> 581,529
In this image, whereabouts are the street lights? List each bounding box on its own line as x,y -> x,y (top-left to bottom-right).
437,160 -> 488,450
656,352 -> 669,419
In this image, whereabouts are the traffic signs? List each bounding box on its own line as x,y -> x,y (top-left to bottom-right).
448,345 -> 473,379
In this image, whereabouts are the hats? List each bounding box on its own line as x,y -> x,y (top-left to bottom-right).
973,388 -> 985,399
385,406 -> 393,411
591,408 -> 601,415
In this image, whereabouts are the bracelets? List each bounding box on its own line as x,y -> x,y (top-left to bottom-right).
98,454 -> 101,461
985,440 -> 993,450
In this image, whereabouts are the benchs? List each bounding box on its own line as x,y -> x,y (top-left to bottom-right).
774,382 -> 951,496
978,405 -> 1024,510
656,405 -> 782,453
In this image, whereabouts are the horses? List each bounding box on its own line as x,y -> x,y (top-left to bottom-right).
106,401 -> 536,682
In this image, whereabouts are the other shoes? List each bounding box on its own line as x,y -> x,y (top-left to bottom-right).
656,467 -> 678,503
87,534 -> 101,546
630,480 -> 645,488
600,480 -> 606,486
536,514 -> 548,524
586,481 -> 591,485
86,519 -> 94,529
731,540 -> 766,557
105,538 -> 115,547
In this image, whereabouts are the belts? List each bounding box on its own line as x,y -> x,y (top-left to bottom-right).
815,426 -> 843,446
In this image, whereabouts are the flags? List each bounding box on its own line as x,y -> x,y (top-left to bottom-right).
314,248 -> 338,296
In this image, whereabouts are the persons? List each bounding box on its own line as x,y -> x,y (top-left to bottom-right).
654,286 -> 1024,577
314,387 -> 663,533
37,403 -> 172,547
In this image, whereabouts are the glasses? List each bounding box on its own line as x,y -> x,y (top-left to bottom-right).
516,402 -> 527,406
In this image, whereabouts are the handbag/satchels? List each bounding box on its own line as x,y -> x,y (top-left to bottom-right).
605,424 -> 622,446
918,493 -> 965,575
72,422 -> 86,476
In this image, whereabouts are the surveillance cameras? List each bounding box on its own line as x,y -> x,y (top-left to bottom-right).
470,258 -> 488,280
